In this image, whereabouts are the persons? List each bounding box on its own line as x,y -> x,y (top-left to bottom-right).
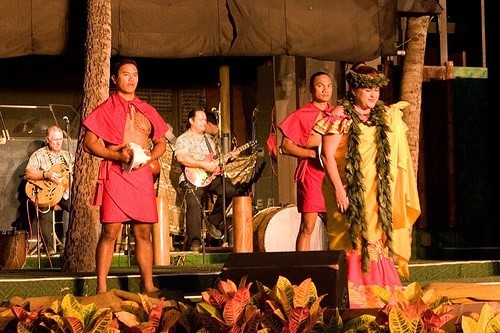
204,112 -> 237,247
25,126 -> 76,255
312,62 -> 421,308
277,72 -> 335,251
83,60 -> 169,295
174,110 -> 236,252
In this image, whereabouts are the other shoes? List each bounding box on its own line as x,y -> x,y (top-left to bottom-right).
47,245 -> 56,255
189,239 -> 201,251
205,220 -> 223,239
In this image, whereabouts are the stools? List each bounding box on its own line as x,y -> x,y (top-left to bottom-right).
182,191 -> 222,251
52,204 -> 62,255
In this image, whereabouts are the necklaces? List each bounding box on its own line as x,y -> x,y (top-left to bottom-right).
48,151 -> 64,166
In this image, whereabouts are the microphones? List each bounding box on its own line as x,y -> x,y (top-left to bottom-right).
211,107 -> 218,113
63,116 -> 69,124
19,174 -> 26,179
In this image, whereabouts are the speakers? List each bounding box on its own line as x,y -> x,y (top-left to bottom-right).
220,249 -> 349,309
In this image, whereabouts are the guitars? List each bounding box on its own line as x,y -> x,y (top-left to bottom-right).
184,138 -> 259,187
24,161 -> 75,209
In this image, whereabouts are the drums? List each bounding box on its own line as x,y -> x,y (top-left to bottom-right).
253,203 -> 329,254
0,229 -> 29,270
251,206 -> 286,247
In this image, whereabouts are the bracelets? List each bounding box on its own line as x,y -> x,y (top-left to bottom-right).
43,171 -> 46,179
